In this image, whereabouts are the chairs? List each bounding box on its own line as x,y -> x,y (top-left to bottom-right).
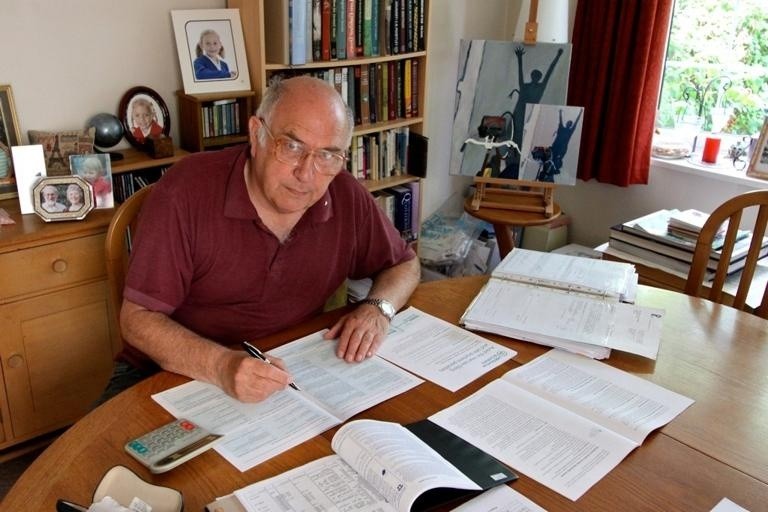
684,189 -> 768,319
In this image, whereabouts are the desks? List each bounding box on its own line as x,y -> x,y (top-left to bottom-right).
464,193 -> 563,262
602,252 -> 757,313
0,277 -> 768,512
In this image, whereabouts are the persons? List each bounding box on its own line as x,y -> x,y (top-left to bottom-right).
194,29 -> 231,79
40,185 -> 67,213
67,184 -> 85,211
96,75 -> 422,407
133,100 -> 163,144
506,45 -> 583,183
82,157 -> 112,207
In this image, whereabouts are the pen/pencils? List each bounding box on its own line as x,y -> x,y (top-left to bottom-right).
242,341 -> 301,391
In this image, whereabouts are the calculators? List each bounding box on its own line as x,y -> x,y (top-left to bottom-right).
124,418 -> 226,475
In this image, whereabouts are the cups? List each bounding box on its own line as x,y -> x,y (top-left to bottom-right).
700,136 -> 721,163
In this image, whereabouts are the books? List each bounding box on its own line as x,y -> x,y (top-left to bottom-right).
202,96 -> 242,139
265,0 -> 425,67
608,208 -> 768,284
346,127 -> 418,243
320,61 -> 422,128
150,246 -> 695,512
114,167 -> 170,205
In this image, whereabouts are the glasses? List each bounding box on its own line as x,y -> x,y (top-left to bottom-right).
259,117 -> 350,177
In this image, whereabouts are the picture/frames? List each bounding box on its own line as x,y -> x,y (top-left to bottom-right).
746,117 -> 768,181
